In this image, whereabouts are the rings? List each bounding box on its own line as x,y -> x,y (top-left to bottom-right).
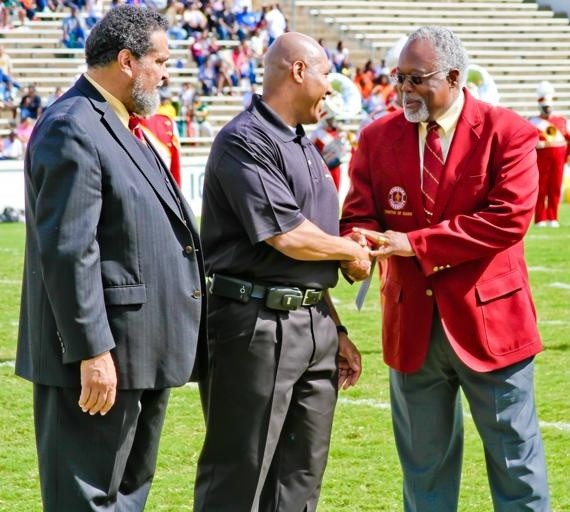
379,236 -> 386,245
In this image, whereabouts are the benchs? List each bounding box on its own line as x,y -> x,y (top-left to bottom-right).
292,0 -> 570,144
1,1 -> 376,144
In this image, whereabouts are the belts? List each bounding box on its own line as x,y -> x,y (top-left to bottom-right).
251,284 -> 326,306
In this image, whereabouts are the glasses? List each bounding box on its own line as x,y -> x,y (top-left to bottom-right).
392,68 -> 448,85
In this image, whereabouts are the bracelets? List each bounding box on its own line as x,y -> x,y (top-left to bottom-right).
337,325 -> 348,336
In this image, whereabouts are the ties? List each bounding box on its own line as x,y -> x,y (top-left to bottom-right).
422,121 -> 445,225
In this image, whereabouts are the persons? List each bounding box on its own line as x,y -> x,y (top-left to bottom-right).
14,7 -> 211,512
157,1 -> 289,138
308,38 -> 400,196
339,24 -> 550,512
1,0 -> 158,159
192,31 -> 374,512
524,80 -> 570,228
